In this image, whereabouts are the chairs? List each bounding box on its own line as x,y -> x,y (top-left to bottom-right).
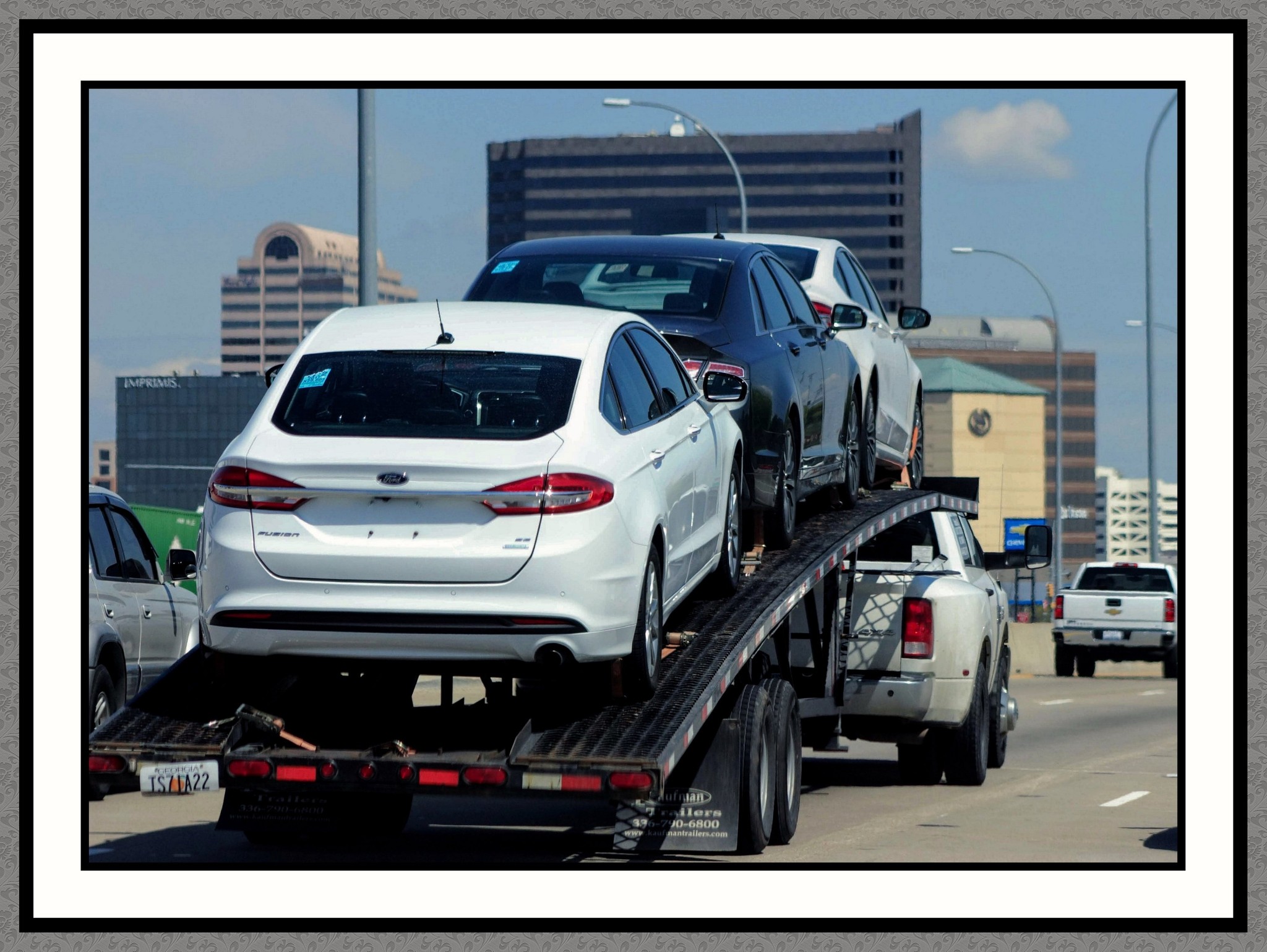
662,293 -> 705,311
542,280 -> 591,305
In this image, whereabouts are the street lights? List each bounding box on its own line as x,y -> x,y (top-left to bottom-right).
601,97 -> 748,233
951,245 -> 1064,596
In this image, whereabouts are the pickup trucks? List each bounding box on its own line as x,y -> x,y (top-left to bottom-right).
1051,560 -> 1177,680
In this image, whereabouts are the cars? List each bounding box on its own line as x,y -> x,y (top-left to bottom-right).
199,297 -> 750,706
462,236 -> 863,551
87,481 -> 199,730
759,506 -> 1052,784
579,231 -> 934,490
